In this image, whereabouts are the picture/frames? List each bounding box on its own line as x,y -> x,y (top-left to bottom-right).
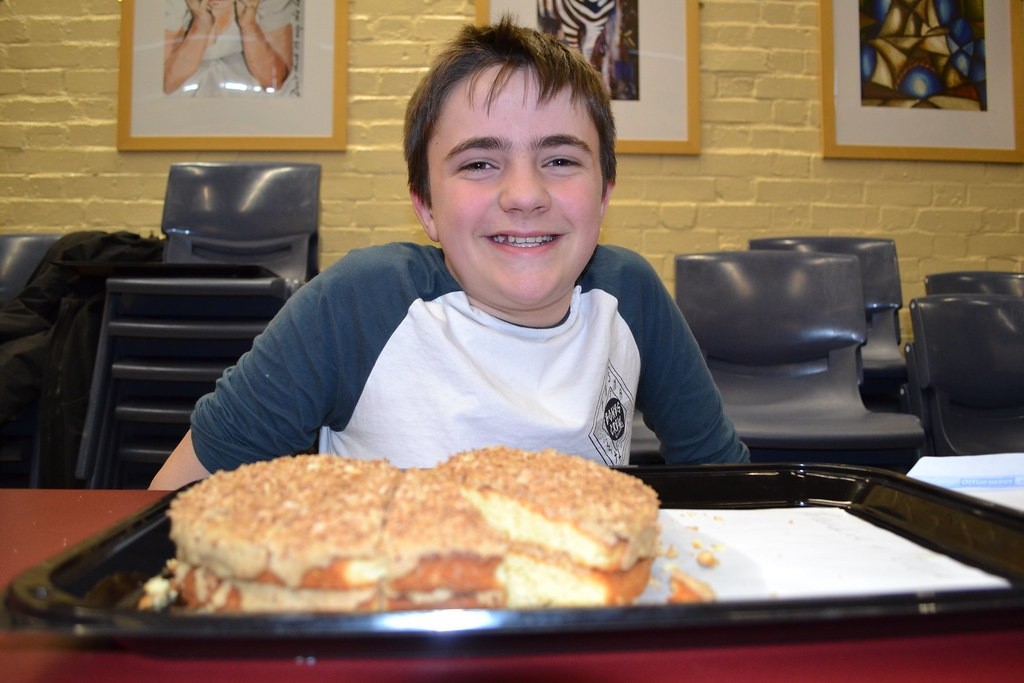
818,0 -> 1024,163
474,0 -> 702,155
117,0 -> 347,152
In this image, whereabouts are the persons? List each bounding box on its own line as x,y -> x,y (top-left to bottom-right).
146,13 -> 755,491
163,0 -> 295,95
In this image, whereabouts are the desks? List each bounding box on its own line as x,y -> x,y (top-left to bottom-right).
0,490 -> 1023,683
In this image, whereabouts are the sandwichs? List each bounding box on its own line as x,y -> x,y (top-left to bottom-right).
166,453 -> 398,612
384,447 -> 661,609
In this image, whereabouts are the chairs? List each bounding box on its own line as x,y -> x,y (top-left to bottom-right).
0,161 -> 321,492
627,236 -> 1024,469
